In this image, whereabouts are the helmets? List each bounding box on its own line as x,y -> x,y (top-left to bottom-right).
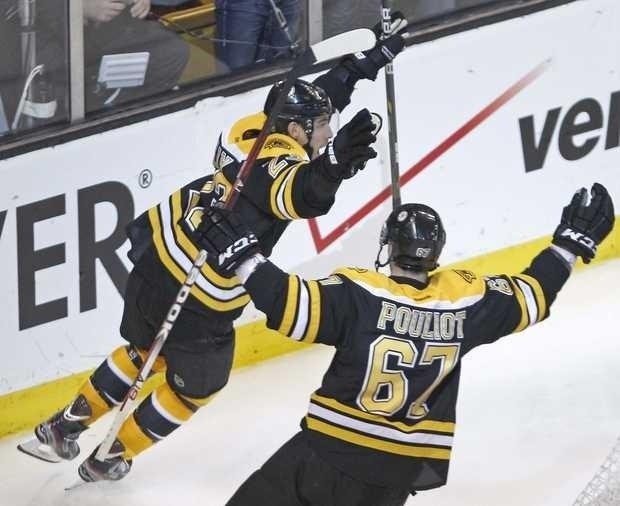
379,203 -> 446,271
264,79 -> 332,120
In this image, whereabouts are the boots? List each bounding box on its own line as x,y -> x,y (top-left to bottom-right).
78,435 -> 132,482
34,394 -> 91,462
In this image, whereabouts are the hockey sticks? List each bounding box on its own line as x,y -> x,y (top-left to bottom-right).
95,27 -> 378,463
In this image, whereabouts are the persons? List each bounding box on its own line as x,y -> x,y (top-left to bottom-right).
30,0 -> 190,122
195,178 -> 620,506
16,11 -> 412,491
214,0 -> 303,73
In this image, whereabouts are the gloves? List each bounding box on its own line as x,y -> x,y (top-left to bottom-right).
341,10 -> 408,81
551,183 -> 615,264
190,206 -> 259,279
319,108 -> 377,180
